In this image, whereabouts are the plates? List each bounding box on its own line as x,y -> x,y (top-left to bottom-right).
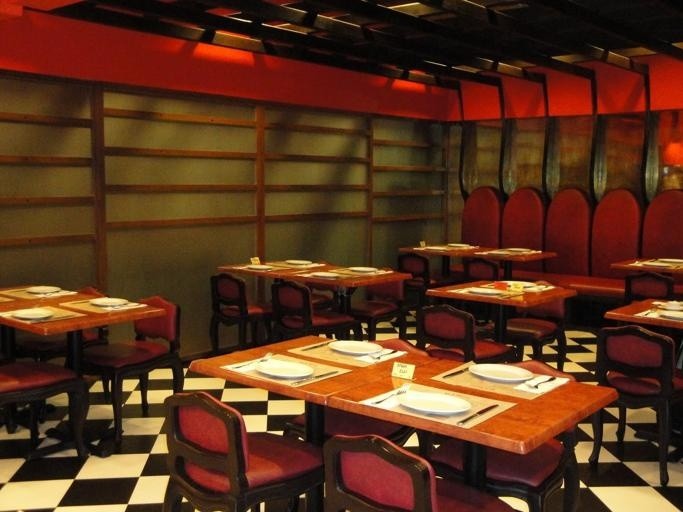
637,261 -> 673,267
11,308 -> 54,319
310,271 -> 342,278
488,249 -> 510,256
469,362 -> 535,384
328,339 -> 384,355
25,285 -> 61,294
447,242 -> 471,248
659,257 -> 683,265
89,296 -> 129,306
349,266 -> 378,272
285,258 -> 313,265
467,287 -> 507,295
253,360 -> 313,380
247,264 -> 275,270
660,309 -> 682,320
424,245 -> 449,251
398,389 -> 472,416
501,279 -> 536,288
505,247 -> 532,252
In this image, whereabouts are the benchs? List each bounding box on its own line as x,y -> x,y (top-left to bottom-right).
448,185 -> 683,327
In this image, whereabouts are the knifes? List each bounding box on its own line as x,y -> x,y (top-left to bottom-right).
291,370 -> 339,385
457,404 -> 500,428
300,339 -> 336,352
442,367 -> 469,380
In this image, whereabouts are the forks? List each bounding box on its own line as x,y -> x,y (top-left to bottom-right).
373,384 -> 406,405
526,376 -> 558,389
369,348 -> 399,361
231,352 -> 274,369
636,304 -> 658,317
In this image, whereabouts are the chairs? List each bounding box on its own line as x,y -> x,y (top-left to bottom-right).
324,433 -> 518,512
307,259 -> 339,310
619,269 -> 674,305
1,360 -> 90,463
12,287 -> 105,364
464,258 -> 497,281
78,297 -> 183,449
588,324 -> 683,486
350,272 -> 413,341
414,302 -> 514,365
428,361 -> 580,512
272,280 -> 358,341
502,293 -> 567,373
397,253 -> 438,336
208,272 -> 272,355
162,392 -> 327,512
396,253 -> 442,307
462,259 -> 498,316
285,340 -> 429,450
